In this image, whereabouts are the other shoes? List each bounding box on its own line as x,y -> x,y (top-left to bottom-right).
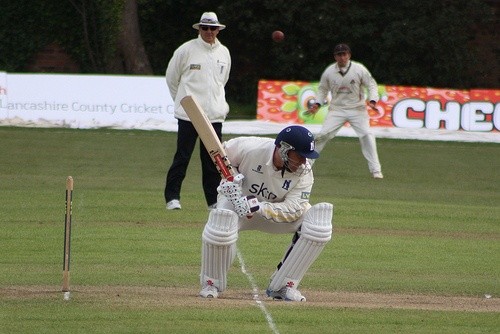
373,172 -> 383,178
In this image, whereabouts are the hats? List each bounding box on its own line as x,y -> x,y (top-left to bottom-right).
334,43 -> 351,54
193,12 -> 226,30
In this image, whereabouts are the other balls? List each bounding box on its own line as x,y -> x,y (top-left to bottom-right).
271,31 -> 284,42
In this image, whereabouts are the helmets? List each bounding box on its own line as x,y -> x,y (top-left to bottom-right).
274,126 -> 320,159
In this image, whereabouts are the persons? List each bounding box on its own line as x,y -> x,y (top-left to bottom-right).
307,42 -> 384,179
164,11 -> 231,210
199,125 -> 334,302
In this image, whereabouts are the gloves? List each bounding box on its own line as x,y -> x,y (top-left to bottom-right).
217,173 -> 244,202
232,195 -> 261,218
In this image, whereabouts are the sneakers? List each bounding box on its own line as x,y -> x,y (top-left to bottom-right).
209,203 -> 217,208
200,285 -> 220,298
266,286 -> 306,302
166,199 -> 181,210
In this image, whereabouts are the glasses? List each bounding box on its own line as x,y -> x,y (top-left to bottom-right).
200,25 -> 219,31
